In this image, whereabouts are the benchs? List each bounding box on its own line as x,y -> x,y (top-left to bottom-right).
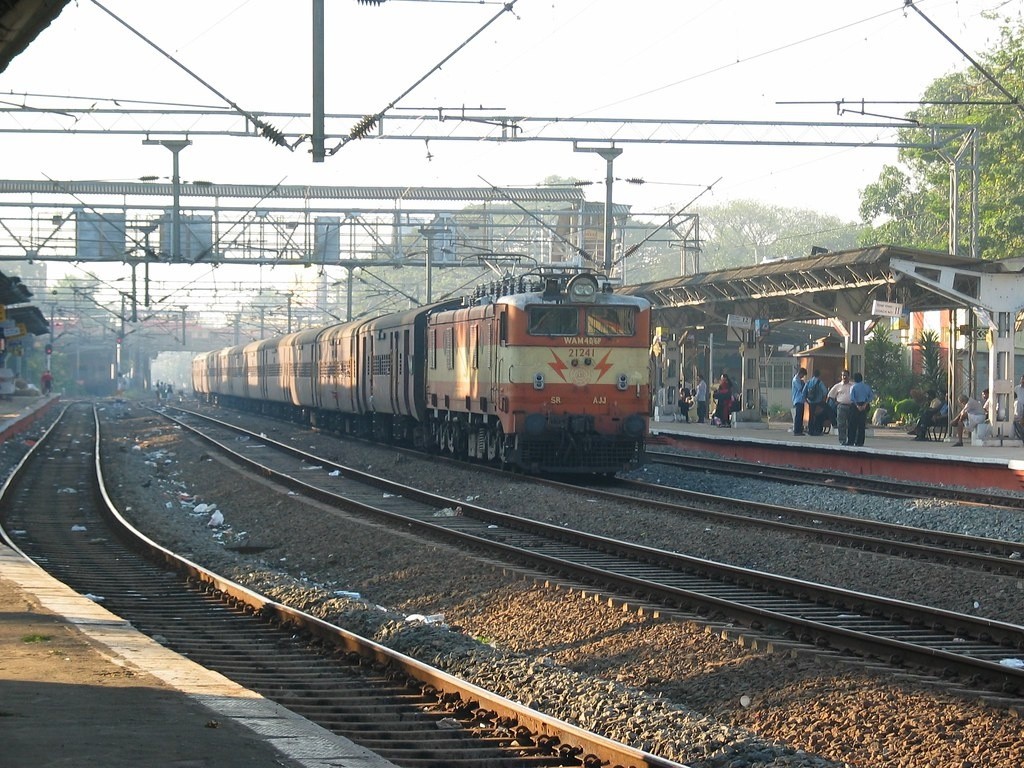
926,419 -> 948,442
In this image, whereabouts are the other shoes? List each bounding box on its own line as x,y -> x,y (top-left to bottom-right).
842,442 -> 854,446
854,443 -> 863,447
953,441 -> 963,446
794,433 -> 805,436
907,430 -> 917,435
949,420 -> 958,427
915,436 -> 925,441
840,441 -> 845,445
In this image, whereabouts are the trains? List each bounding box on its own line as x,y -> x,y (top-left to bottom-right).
191,265 -> 654,475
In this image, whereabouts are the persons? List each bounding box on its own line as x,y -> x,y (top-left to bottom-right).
41,370 -> 54,395
792,368 -> 873,447
153,380 -> 174,407
678,374 -> 741,428
907,375 -> 1024,447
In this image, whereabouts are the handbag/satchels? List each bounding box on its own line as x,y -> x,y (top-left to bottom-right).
718,393 -> 729,400
807,383 -> 820,401
730,394 -> 740,412
